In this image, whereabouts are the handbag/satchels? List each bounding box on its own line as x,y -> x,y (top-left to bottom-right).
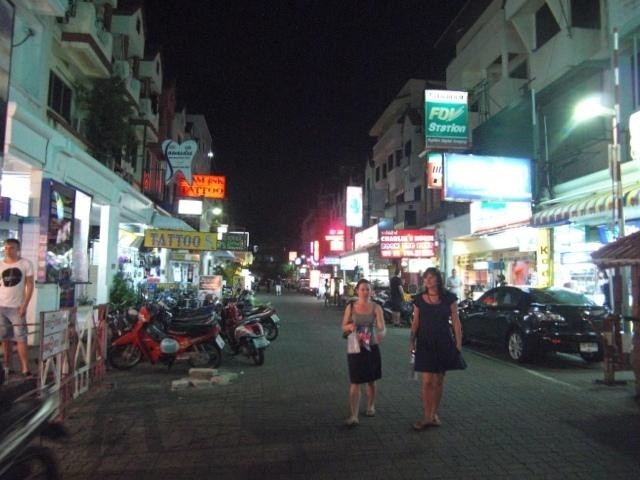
342,320 -> 355,339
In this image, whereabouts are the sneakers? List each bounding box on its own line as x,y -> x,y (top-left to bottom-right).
414,414 -> 441,429
346,408 -> 375,425
4,368 -> 31,378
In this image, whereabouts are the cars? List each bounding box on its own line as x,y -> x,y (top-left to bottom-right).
449,285 -> 612,364
297,279 -> 310,293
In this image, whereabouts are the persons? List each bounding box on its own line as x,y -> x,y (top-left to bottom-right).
333,279 -> 341,306
448,269 -> 463,305
597,270 -> 610,309
256,274 -> 288,296
0,239 -> 34,378
300,272 -> 305,279
226,282 -> 232,290
325,279 -> 332,308
236,278 -> 241,294
390,270 -> 407,328
340,279 -> 387,427
407,267 -> 464,430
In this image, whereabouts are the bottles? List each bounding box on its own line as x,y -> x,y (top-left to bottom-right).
407,349 -> 418,382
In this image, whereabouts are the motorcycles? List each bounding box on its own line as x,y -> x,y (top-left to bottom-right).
107,286 -> 279,369
374,289 -> 474,329
